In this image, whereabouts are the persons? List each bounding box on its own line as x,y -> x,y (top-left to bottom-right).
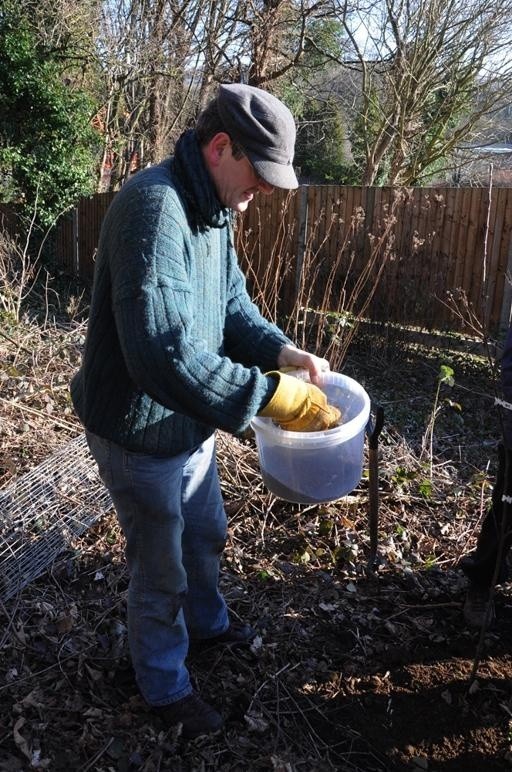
70,82 -> 340,740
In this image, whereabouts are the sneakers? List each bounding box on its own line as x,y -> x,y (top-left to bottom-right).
153,688 -> 223,740
189,624 -> 252,642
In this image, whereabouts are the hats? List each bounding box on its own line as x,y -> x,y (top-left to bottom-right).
216,83 -> 299,190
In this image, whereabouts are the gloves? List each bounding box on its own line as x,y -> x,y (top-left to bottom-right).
255,370 -> 342,432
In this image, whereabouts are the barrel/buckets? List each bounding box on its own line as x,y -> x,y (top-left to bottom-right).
248,369 -> 370,504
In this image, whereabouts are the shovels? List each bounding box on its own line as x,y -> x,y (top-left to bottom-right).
364,402 -> 385,590
